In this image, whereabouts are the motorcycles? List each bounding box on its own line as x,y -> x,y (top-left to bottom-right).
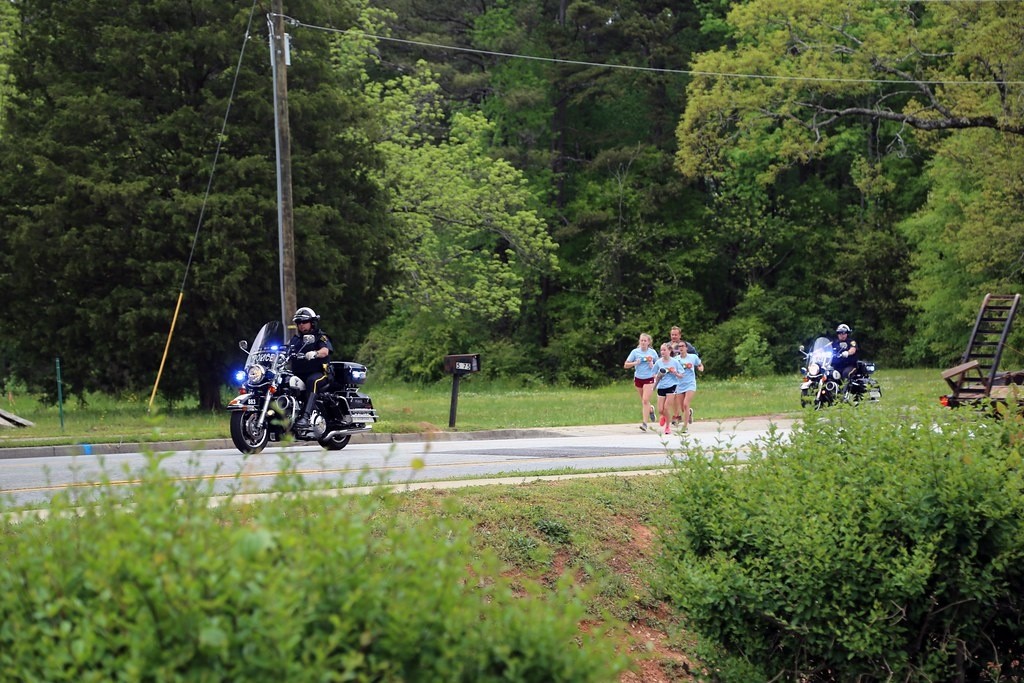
226,319 -> 380,454
799,337 -> 882,411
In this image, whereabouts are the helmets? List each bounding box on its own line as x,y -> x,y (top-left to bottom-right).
836,323 -> 852,334
292,306 -> 321,322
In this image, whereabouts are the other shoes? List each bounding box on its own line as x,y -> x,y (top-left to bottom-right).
689,408 -> 693,424
682,427 -> 688,433
672,415 -> 683,425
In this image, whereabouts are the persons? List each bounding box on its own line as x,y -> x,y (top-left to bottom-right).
279,305 -> 334,430
667,324 -> 699,426
651,342 -> 686,434
622,330 -> 660,432
673,341 -> 704,434
807,323 -> 859,405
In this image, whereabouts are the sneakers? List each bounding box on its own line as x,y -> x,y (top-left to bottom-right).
660,414 -> 665,426
639,422 -> 647,431
649,405 -> 656,422
665,427 -> 671,434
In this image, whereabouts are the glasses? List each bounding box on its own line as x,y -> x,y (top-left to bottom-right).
295,320 -> 310,324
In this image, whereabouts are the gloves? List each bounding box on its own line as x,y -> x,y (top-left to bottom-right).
305,350 -> 318,361
842,350 -> 848,358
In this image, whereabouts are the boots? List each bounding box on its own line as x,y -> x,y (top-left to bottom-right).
296,392 -> 317,426
